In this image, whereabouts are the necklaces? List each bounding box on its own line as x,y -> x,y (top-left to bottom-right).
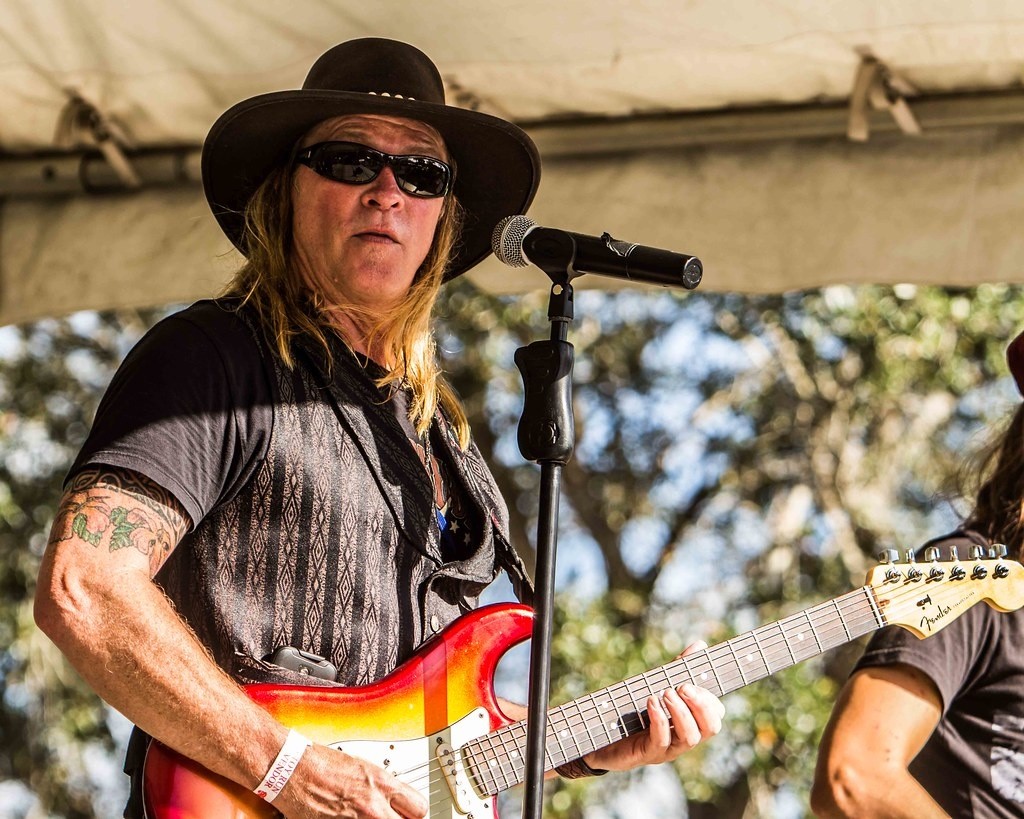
383,386 -> 451,533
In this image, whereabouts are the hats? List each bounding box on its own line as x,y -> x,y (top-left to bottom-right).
202,38 -> 542,288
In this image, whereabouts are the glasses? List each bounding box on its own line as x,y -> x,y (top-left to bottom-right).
290,141 -> 452,199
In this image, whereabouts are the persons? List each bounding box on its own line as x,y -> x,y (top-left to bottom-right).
809,330 -> 1023,819
32,40 -> 727,817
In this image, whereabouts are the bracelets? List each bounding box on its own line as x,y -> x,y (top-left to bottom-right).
254,727 -> 313,805
554,757 -> 609,780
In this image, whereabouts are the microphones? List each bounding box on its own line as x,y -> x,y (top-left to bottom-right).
491,214 -> 704,290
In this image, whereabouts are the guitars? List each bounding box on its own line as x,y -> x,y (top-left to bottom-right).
139,544 -> 1024,819
